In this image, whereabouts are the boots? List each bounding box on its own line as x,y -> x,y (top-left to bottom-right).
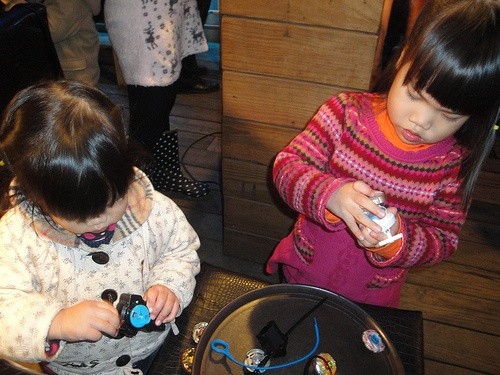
151,128 -> 210,199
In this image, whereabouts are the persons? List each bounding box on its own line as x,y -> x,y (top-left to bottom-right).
0,0 -> 102,86
181,0 -> 220,92
0,81 -> 202,374
266,0 -> 499,308
103,0 -> 212,201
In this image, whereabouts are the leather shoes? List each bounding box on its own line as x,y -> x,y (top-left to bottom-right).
175,78 -> 220,94
196,64 -> 208,75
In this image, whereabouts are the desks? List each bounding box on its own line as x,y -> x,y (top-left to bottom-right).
145,261 -> 425,375
